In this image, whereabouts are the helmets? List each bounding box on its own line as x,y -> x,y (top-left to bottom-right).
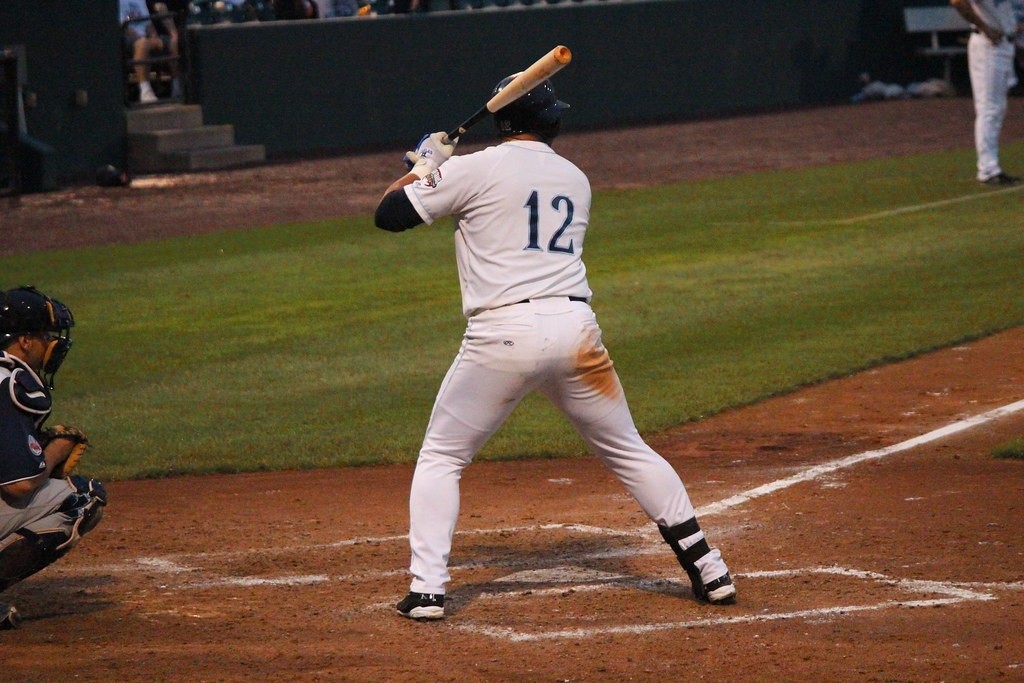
490,73 -> 570,138
0,284 -> 55,338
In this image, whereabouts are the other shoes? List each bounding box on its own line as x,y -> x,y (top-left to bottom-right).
0,601 -> 21,629
984,173 -> 1021,186
140,87 -> 158,104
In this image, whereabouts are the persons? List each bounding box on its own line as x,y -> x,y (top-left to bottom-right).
0,285 -> 108,628
950,1 -> 1024,187
119,0 -> 489,102
372,71 -> 738,619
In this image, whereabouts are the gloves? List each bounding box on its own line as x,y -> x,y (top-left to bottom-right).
402,131 -> 461,181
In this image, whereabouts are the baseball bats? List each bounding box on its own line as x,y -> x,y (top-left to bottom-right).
441,43 -> 572,146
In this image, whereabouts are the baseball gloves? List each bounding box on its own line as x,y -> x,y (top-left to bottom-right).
39,425 -> 87,479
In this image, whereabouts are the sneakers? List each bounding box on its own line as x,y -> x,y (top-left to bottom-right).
396,592 -> 445,619
702,573 -> 735,602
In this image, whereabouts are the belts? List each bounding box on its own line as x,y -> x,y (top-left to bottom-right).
517,294 -> 587,303
970,27 -> 1013,42
